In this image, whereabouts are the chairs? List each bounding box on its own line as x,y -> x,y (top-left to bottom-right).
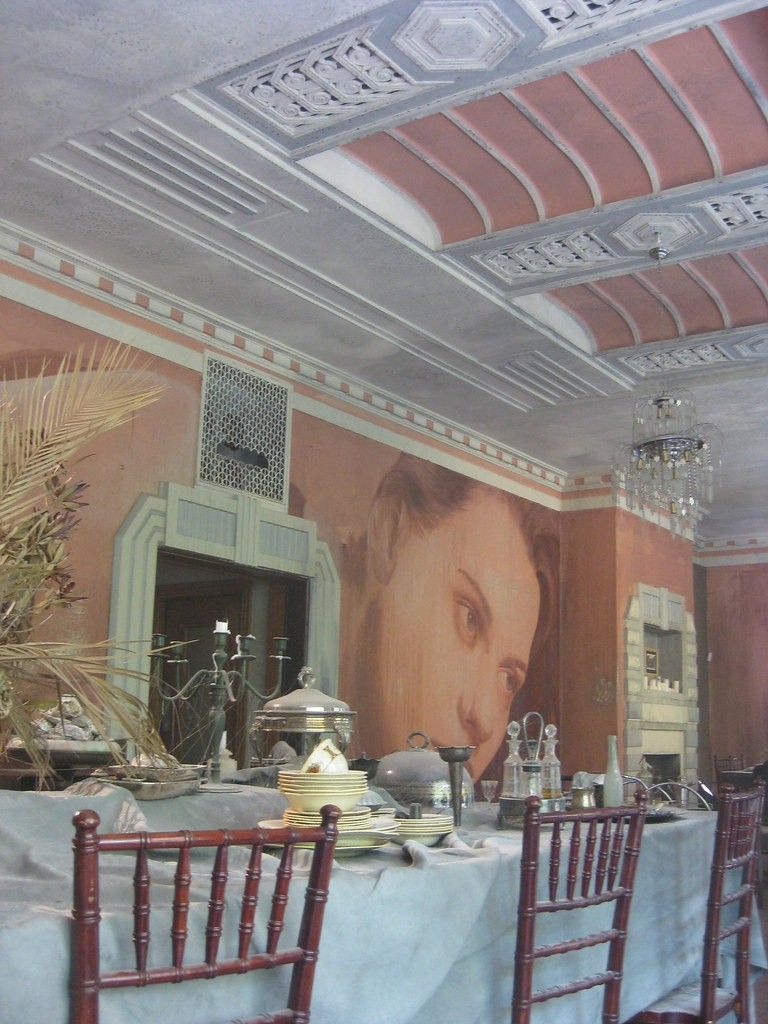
638,763 -> 767,1023
67,802 -> 344,1023
713,755 -> 732,795
510,787 -> 648,1022
732,754 -> 744,771
584,900 -> 588,904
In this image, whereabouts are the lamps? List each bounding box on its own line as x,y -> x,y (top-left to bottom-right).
609,231 -> 729,534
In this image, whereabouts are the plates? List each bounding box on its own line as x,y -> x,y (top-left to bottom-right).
258,806 -> 400,858
97,762 -> 208,800
640,804 -> 688,820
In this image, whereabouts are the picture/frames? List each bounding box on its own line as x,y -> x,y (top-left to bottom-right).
645,651 -> 657,672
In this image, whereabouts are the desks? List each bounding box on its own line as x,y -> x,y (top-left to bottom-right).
1,780 -> 768,1023
721,765 -> 755,793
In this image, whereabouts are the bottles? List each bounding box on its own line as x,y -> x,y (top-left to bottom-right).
570,787 -> 596,810
206,749 -> 238,784
603,735 -> 623,808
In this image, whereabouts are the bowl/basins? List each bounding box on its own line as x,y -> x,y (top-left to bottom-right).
275,769 -> 369,811
391,813 -> 454,846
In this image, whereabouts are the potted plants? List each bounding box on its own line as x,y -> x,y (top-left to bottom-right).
0,333 -> 180,791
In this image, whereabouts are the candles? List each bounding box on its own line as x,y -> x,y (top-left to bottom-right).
215,618 -> 231,632
219,730 -> 228,749
